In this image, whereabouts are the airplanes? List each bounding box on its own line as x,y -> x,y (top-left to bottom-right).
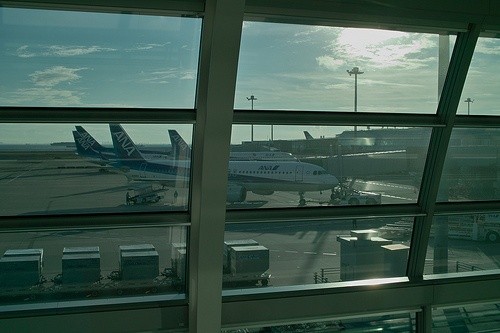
72,123 -> 340,206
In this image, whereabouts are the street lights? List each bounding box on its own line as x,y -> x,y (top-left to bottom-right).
246,95 -> 258,143
346,66 -> 365,153
464,97 -> 475,115
